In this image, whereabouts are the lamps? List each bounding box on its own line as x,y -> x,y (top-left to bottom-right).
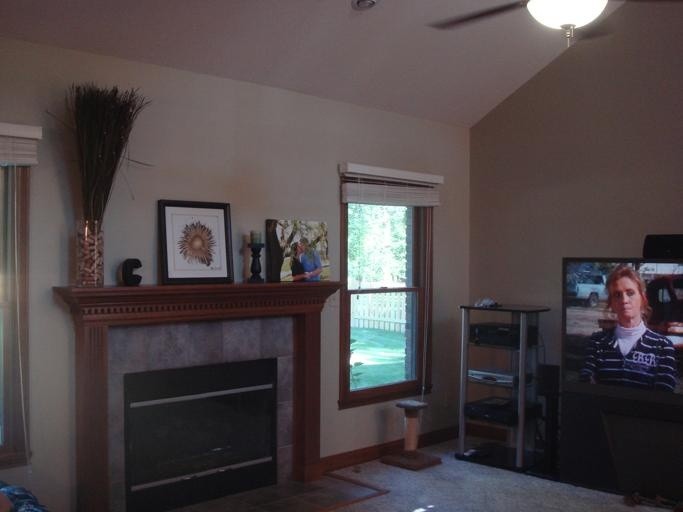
522,0 -> 610,46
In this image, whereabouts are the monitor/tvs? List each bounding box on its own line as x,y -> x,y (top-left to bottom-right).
561,256 -> 683,424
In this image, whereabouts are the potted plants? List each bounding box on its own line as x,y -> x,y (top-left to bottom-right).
45,83 -> 150,288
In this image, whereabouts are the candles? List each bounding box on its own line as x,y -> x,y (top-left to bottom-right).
250,230 -> 262,243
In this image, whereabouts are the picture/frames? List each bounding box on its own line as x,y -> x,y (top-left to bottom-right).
159,195 -> 234,285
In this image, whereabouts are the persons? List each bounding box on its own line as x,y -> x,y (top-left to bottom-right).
300,238 -> 323,281
581,269 -> 677,393
291,242 -> 311,282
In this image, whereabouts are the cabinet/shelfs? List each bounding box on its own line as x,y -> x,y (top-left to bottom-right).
459,302 -> 550,471
554,396 -> 683,503
